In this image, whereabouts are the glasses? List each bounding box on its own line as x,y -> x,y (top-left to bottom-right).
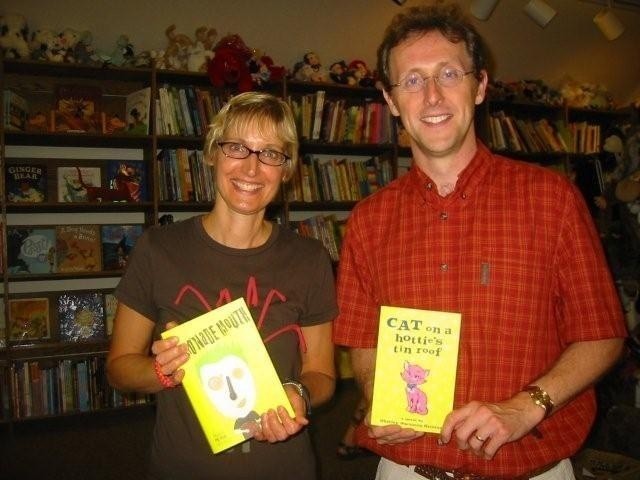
217,141 -> 291,166
389,69 -> 476,92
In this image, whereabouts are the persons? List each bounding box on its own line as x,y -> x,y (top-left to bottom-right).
330,5 -> 628,480
106,91 -> 340,480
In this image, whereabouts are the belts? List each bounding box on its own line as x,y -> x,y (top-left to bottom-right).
405,463 -> 488,479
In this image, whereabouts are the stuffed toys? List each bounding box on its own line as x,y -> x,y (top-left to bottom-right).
286,24 -> 387,87
156,20 -> 291,95
592,133 -> 640,222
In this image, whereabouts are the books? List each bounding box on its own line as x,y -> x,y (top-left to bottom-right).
0,87 -> 151,418
155,83 -> 390,263
370,307 -> 463,434
487,109 -> 602,153
161,296 -> 295,453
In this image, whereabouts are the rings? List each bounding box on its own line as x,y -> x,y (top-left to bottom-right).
474,432 -> 486,444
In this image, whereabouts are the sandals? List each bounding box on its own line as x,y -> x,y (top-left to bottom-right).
337,442 -> 372,460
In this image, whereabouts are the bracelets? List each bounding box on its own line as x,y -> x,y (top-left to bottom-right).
281,377 -> 313,415
153,360 -> 175,392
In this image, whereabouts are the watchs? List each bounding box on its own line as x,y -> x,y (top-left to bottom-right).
523,384 -> 555,415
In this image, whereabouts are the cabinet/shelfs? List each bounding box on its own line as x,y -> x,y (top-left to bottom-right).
1,56 -> 157,425
486,96 -> 639,410
156,59 -> 291,232
396,92 -> 490,165
282,65 -> 398,388
